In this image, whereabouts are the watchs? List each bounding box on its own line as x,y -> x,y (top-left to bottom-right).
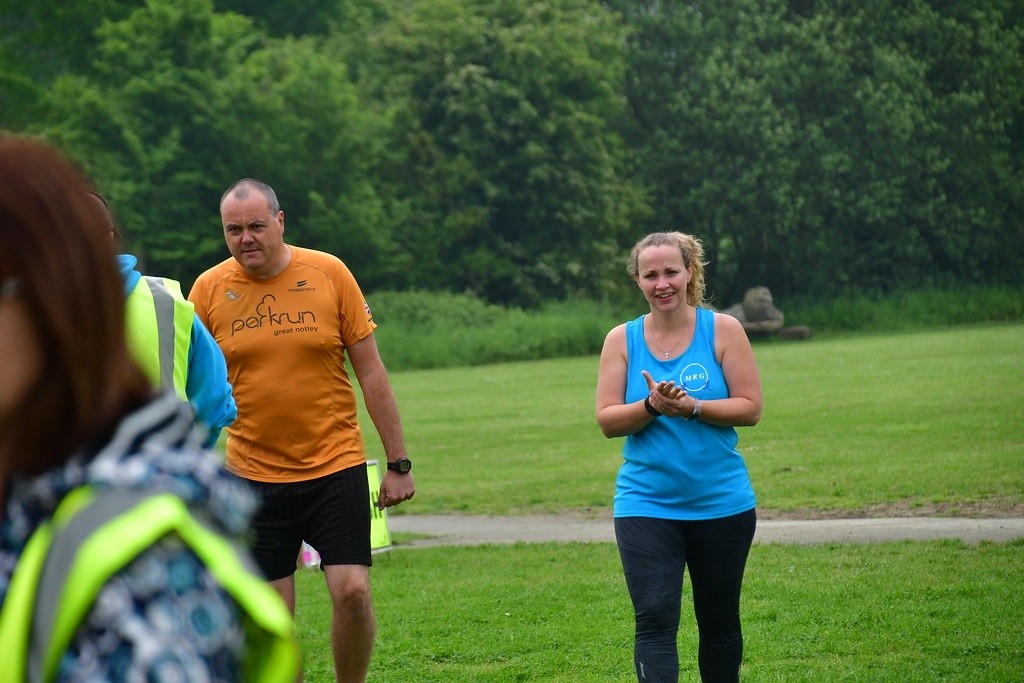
685,397 -> 701,421
387,457 -> 411,474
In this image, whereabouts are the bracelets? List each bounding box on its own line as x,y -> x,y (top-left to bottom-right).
644,395 -> 663,416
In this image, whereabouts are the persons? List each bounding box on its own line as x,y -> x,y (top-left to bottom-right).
596,232 -> 765,683
0,134 -> 306,683
183,178 -> 415,683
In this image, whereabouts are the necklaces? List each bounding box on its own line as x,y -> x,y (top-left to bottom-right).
650,312 -> 682,359
